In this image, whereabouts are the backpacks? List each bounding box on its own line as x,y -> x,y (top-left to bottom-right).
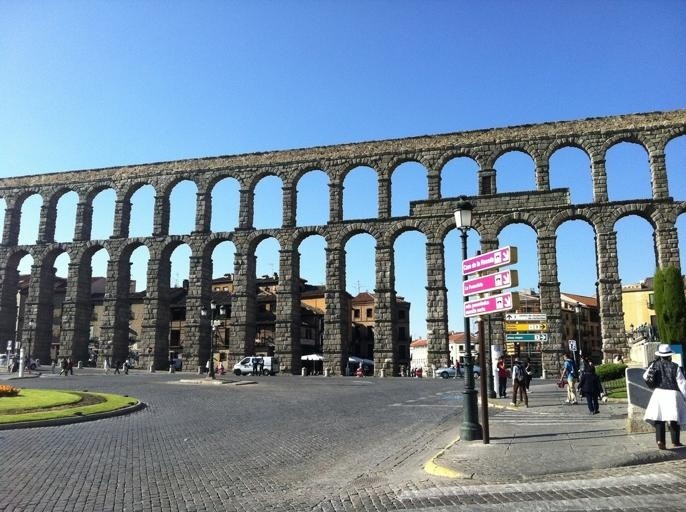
566,360 -> 580,378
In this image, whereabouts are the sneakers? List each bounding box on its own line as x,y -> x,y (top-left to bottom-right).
500,390 -> 578,407
658,440 -> 685,450
590,410 -> 601,415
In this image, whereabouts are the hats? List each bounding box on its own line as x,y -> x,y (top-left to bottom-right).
655,343 -> 674,356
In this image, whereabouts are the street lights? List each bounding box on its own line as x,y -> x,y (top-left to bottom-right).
29,319 -> 34,370
201,299 -> 226,376
453,197 -> 483,441
575,302 -> 582,362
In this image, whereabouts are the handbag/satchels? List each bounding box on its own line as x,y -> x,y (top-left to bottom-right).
643,363 -> 661,390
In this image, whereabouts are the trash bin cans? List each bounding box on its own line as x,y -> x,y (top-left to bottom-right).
301,367 -> 307,377
150,365 -> 155,373
35,359 -> 40,367
325,368 -> 331,376
78,360 -> 82,369
381,369 -> 385,377
197,366 -> 203,374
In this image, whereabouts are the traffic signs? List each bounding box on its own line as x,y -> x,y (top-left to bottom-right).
462,245 -> 520,318
505,313 -> 550,343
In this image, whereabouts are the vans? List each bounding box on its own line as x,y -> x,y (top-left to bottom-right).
232,357 -> 280,376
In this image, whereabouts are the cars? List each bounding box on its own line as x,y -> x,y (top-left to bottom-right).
435,363 -> 481,379
0,354 -> 38,370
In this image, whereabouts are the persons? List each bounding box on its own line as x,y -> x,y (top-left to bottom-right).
215,365 -> 224,372
453,359 -> 463,377
559,353 -> 578,405
400,365 -> 406,377
630,321 -> 649,340
524,362 -> 534,393
60,357 -> 73,376
104,358 -> 129,376
577,364 -> 603,416
11,355 -> 18,372
511,357 -> 530,409
206,359 -> 210,374
496,354 -> 510,398
24,356 -> 29,369
252,357 -> 264,376
643,343 -> 686,450
